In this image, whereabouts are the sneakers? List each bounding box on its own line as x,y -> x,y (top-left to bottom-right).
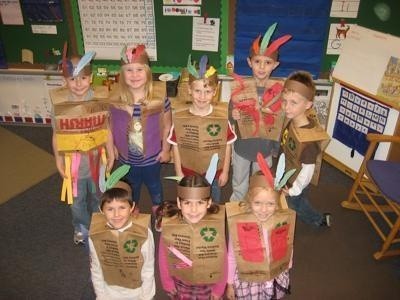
151,206 -> 163,232
72,230 -> 88,249
320,213 -> 334,228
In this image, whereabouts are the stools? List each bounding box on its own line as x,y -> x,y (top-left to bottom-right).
341,134 -> 400,260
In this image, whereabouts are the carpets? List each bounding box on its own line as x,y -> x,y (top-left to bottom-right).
0,125 -> 59,206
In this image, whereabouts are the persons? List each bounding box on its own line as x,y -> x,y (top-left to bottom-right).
169,63 -> 237,205
108,44 -> 174,233
155,174 -> 228,300
272,72 -> 335,234
50,56 -> 116,246
88,180 -> 156,300
225,170 -> 293,300
227,40 -> 287,205
